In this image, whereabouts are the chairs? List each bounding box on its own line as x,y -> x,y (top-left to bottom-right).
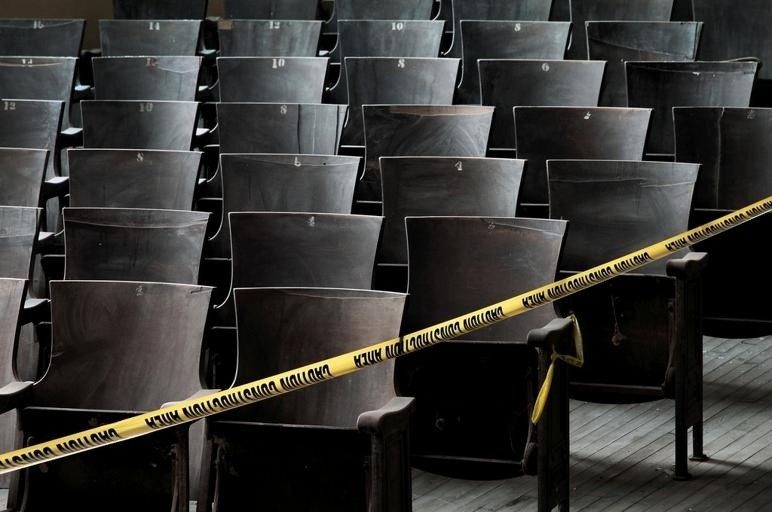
1,1 -> 772,511
